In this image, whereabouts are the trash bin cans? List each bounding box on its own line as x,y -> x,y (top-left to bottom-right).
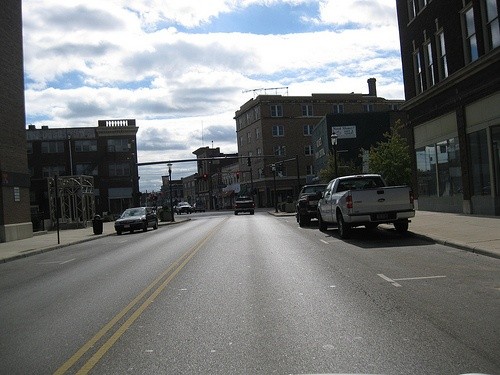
92,215 -> 103,234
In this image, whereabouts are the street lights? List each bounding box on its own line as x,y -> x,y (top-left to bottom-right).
330,132 -> 341,178
272,163 -> 279,212
167,163 -> 175,221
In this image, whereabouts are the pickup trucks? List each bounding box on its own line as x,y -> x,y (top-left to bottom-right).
315,174 -> 419,237
296,184 -> 328,226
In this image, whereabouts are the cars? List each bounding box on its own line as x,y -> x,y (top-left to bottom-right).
114,207 -> 159,234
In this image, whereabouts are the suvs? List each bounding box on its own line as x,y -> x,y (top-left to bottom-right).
174,201 -> 206,213
234,197 -> 255,214
